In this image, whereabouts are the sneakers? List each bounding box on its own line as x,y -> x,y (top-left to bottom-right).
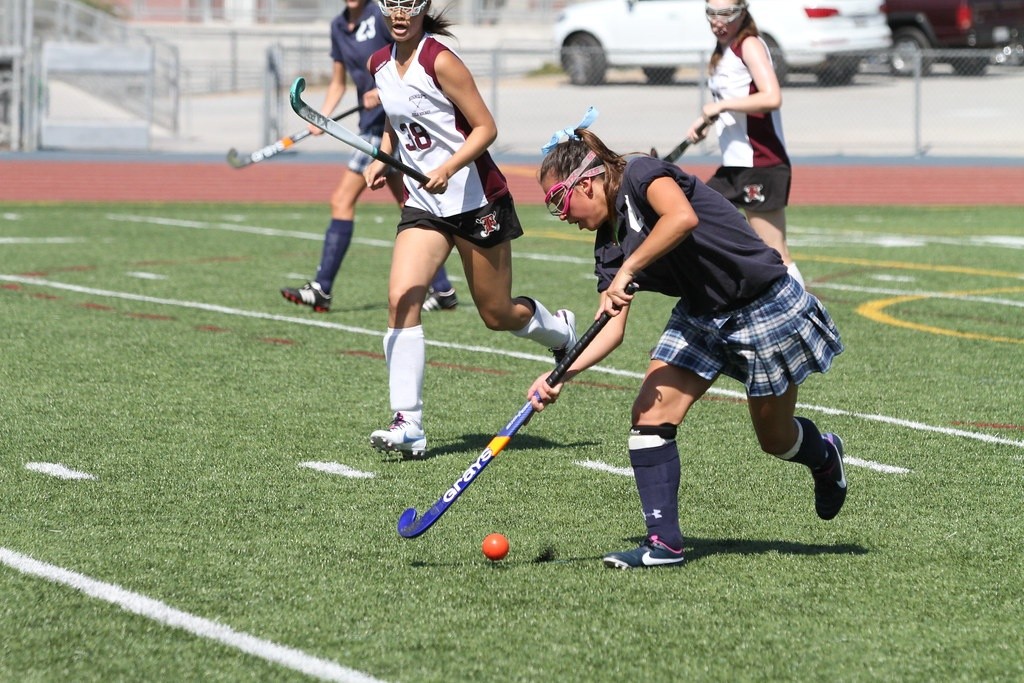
422,288 -> 458,312
280,281 -> 330,313
548,309 -> 578,367
603,535 -> 685,568
370,412 -> 426,457
815,432 -> 847,520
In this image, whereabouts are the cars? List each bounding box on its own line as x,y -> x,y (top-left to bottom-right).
544,0 -> 895,90
883,0 -> 1024,77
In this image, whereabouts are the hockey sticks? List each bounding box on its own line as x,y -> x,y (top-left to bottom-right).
650,112 -> 720,165
225,105 -> 366,170
289,76 -> 431,186
396,278 -> 641,541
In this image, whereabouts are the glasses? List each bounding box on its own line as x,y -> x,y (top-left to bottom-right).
704,4 -> 748,24
546,148 -> 605,216
374,0 -> 428,17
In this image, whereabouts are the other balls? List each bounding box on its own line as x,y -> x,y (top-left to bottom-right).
483,531 -> 510,561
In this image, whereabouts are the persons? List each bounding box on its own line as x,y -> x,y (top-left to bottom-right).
685,0 -> 806,290
362,1 -> 577,460
279,1 -> 458,314
526,107 -> 847,570
